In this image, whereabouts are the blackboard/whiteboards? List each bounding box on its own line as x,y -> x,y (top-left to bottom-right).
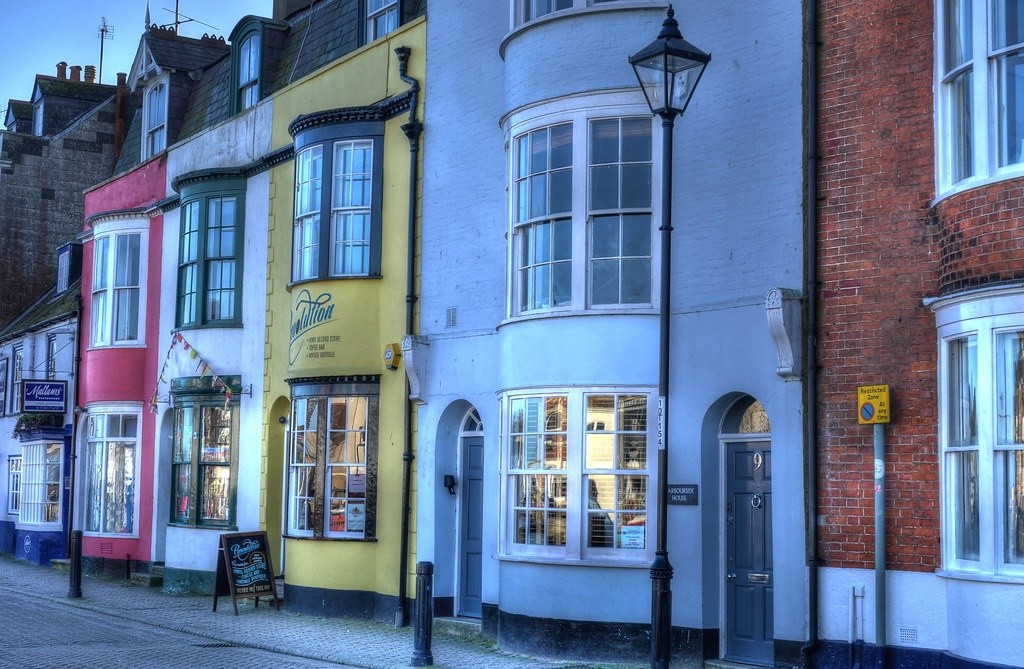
221,531 -> 278,598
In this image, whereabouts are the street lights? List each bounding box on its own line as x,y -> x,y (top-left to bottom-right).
627,2 -> 711,664
